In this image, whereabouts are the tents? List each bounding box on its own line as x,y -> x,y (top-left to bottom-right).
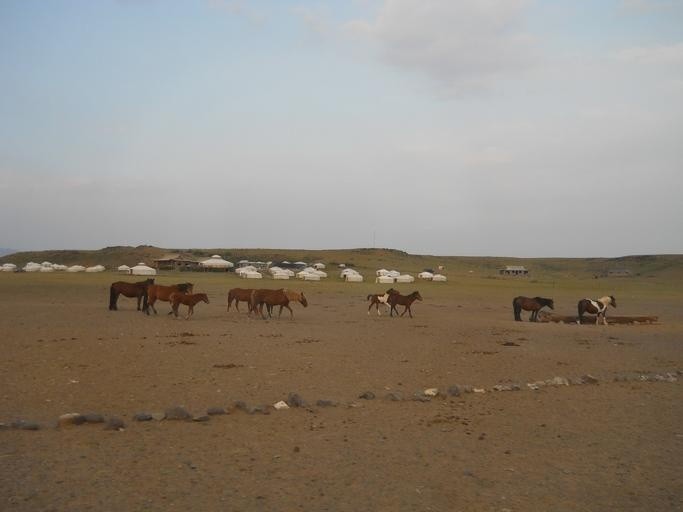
235,258 -> 446,284
0,260 -> 156,276
198,254 -> 234,273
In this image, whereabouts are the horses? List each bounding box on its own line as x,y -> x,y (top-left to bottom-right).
366,287 -> 399,315
227,288 -> 307,319
169,292 -> 208,319
143,282 -> 194,316
575,295 -> 616,325
386,290 -> 423,318
108,277 -> 154,311
512,295 -> 553,322
